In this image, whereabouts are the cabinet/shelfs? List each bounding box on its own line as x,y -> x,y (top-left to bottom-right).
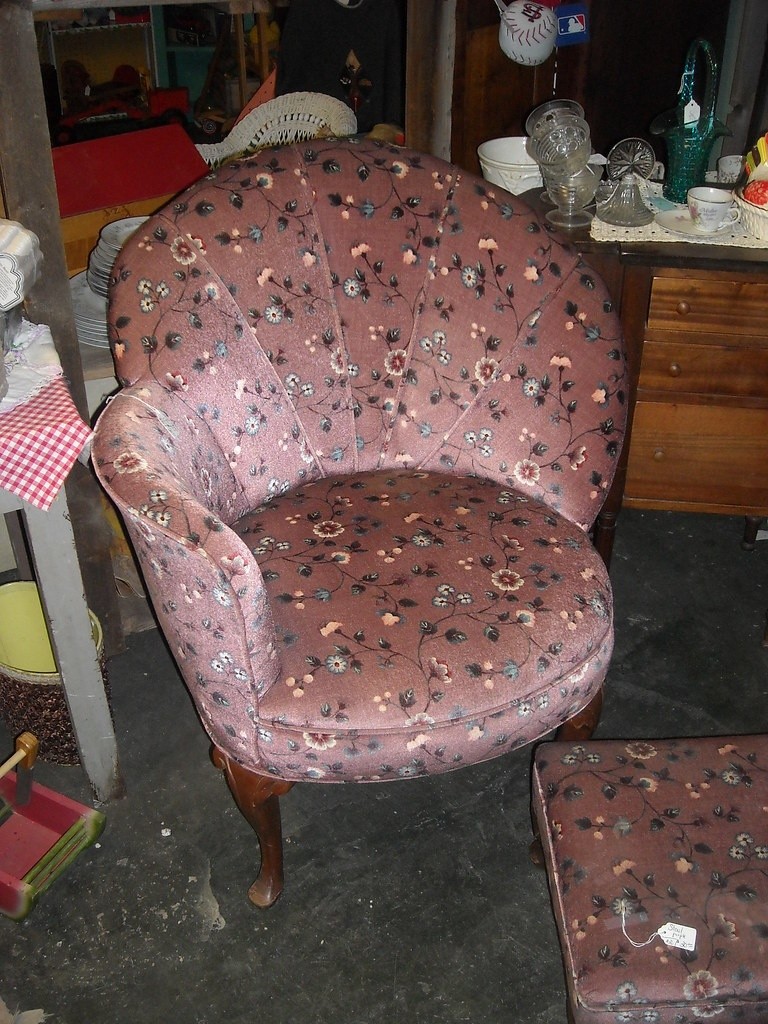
517,186 -> 767,555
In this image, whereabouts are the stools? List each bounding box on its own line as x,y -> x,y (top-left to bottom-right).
525,732 -> 768,1023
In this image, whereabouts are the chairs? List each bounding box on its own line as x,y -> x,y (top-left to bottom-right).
92,136 -> 630,907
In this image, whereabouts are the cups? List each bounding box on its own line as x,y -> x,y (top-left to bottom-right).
526,100 -> 603,227
687,187 -> 741,233
718,155 -> 747,184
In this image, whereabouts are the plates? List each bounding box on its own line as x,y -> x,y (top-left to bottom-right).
69,270 -> 111,348
87,216 -> 152,300
654,209 -> 732,237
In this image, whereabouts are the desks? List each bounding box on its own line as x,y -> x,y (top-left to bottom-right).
0,326 -> 127,805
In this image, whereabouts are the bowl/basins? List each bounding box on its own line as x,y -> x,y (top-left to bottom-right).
478,137 -> 545,196
